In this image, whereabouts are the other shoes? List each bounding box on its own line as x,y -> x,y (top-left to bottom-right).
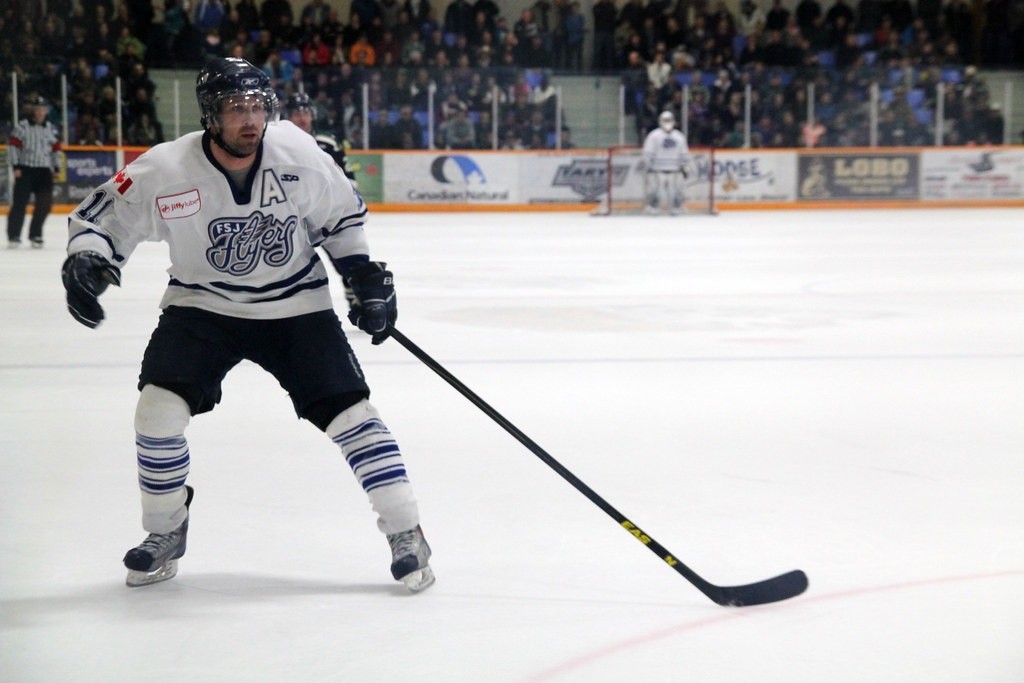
32,236 -> 44,247
10,240 -> 21,246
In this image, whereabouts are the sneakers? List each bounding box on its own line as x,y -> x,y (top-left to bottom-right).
386,524 -> 435,591
123,485 -> 194,587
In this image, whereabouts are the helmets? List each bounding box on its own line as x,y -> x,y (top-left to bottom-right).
659,111 -> 675,131
284,92 -> 312,109
32,96 -> 49,106
195,56 -> 280,131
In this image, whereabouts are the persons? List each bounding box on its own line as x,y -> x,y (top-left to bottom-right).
635,111 -> 696,216
283,92 -> 359,192
61,57 -> 432,582
0,0 -> 1004,150
7,97 -> 61,246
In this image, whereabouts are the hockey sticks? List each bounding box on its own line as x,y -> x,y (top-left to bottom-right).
386,324 -> 810,610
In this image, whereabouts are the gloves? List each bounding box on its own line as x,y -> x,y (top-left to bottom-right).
61,251 -> 121,329
343,261 -> 397,345
